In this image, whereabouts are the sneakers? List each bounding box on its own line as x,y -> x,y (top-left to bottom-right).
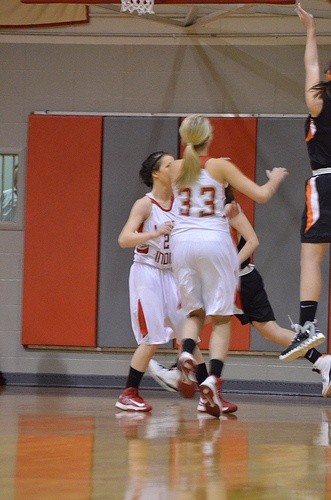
279,314 -> 326,363
114,387 -> 152,412
199,375 -> 224,418
197,395 -> 238,413
312,353 -> 331,398
148,359 -> 181,393
178,351 -> 197,399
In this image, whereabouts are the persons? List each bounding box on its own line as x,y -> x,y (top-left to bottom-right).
279,1 -> 331,362
116,150 -> 239,413
145,183 -> 331,399
165,113 -> 290,419
